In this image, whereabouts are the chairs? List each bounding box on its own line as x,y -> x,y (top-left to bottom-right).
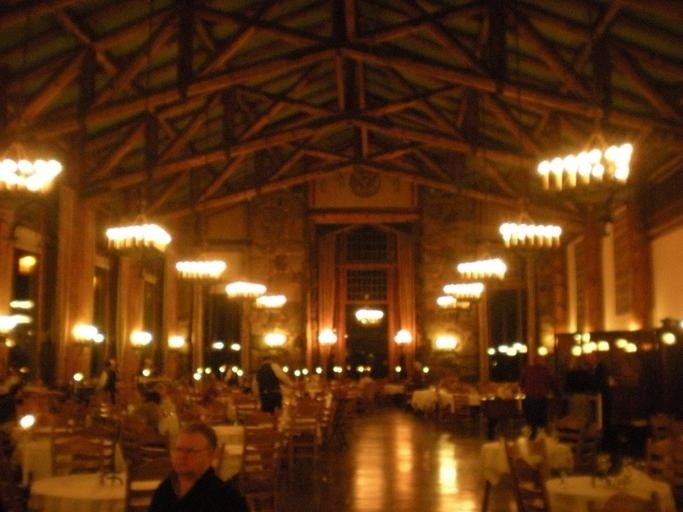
405,377 -> 683,511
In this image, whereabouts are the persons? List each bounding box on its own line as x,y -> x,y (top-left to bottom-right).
94,358 -> 115,397
404,360 -> 428,390
251,353 -> 298,416
133,356 -> 160,404
144,421 -> 253,512
516,355 -> 562,433
563,359 -> 619,454
